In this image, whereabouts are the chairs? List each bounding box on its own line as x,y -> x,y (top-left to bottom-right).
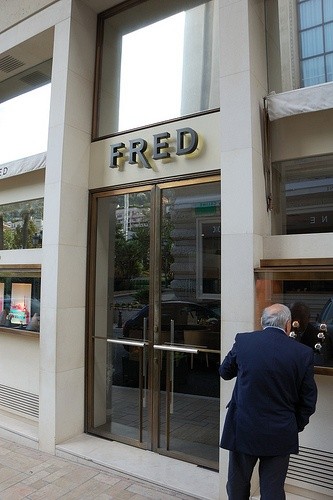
129,328 -> 209,369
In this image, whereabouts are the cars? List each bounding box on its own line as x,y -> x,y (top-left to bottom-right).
123,300 -> 221,355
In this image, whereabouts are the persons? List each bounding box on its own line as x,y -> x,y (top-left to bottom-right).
289,300 -> 323,349
218,303 -> 318,499
